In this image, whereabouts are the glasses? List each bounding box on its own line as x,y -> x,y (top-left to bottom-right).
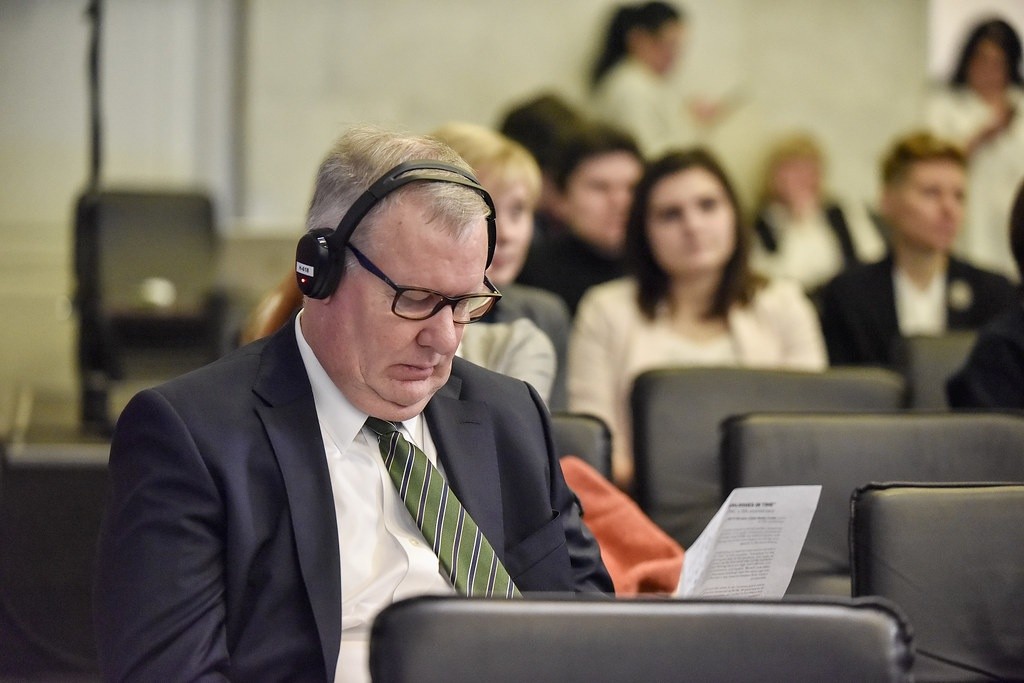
346,242 -> 503,325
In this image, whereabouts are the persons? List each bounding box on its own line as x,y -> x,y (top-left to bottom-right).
104,135 -> 615,681
429,1 -> 1019,417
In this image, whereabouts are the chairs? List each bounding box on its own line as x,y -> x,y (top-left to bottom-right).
849,482 -> 1024,683
370,596 -> 916,683
0,444 -> 109,683
721,413 -> 1024,594
74,192 -> 240,443
633,366 -> 907,550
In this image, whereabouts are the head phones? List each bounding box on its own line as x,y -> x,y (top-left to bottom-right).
295,159 -> 497,300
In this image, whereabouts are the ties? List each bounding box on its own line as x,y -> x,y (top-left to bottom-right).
368,415 -> 520,602
